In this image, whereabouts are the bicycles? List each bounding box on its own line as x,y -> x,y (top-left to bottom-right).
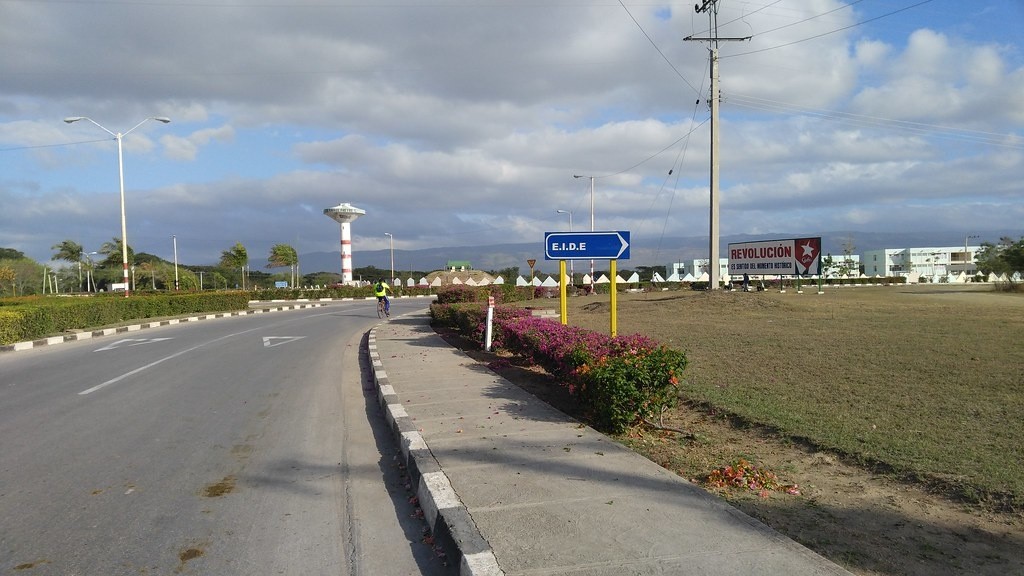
378,291 -> 393,319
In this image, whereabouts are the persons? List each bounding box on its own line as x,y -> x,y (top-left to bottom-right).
756,281 -> 764,291
374,278 -> 393,316
743,272 -> 749,291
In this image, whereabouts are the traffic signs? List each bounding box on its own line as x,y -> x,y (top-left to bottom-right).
545,229 -> 630,260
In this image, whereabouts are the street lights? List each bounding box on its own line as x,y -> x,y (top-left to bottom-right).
63,115 -> 172,299
557,211 -> 574,286
385,232 -> 394,289
78,251 -> 97,294
574,174 -> 596,294
